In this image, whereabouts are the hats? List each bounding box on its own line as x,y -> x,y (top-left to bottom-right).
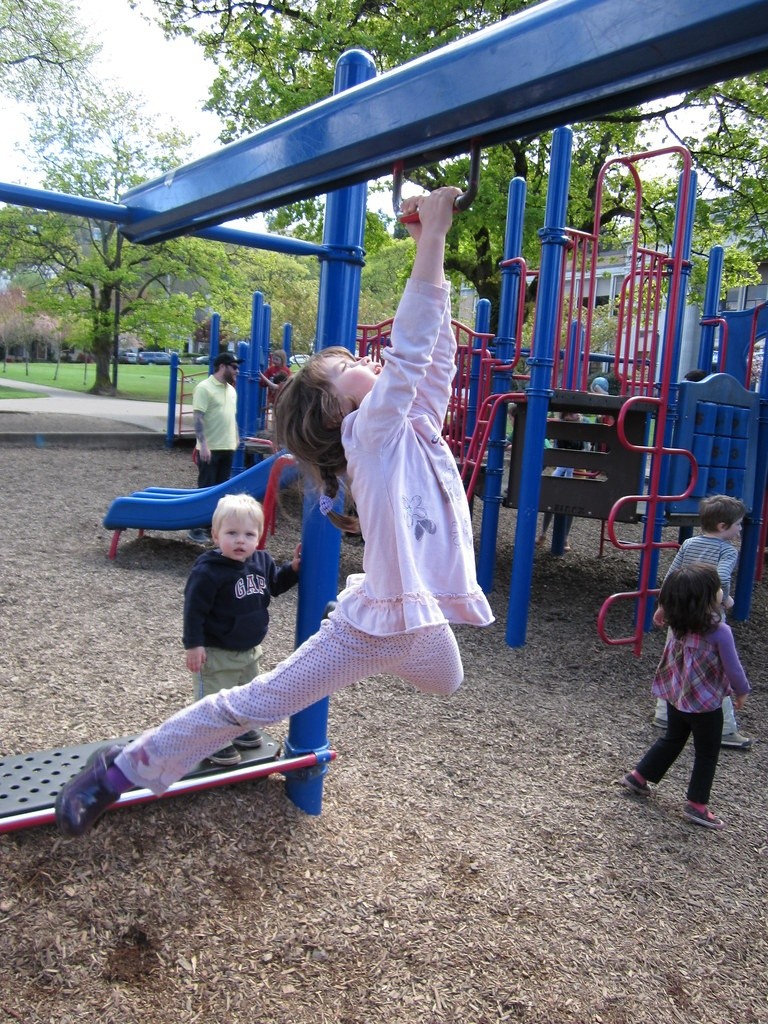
213,352 -> 245,366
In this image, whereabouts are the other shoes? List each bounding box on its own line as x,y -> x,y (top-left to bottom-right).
564,541 -> 570,551
234,728 -> 263,748
721,734 -> 753,748
208,744 -> 240,764
536,535 -> 546,545
622,772 -> 651,795
55,742 -> 127,838
653,716 -> 669,729
684,804 -> 725,829
187,528 -> 209,542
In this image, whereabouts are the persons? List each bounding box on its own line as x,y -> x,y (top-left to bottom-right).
534,408 -> 602,555
55,188 -> 495,840
188,351 -> 246,544
260,349 -> 291,403
652,494 -> 755,749
623,563 -> 752,829
182,494 -> 303,767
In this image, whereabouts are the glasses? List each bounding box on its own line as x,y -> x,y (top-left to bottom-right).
226,363 -> 239,370
272,357 -> 282,361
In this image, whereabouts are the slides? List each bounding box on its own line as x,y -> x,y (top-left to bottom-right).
103,447 -> 306,530
